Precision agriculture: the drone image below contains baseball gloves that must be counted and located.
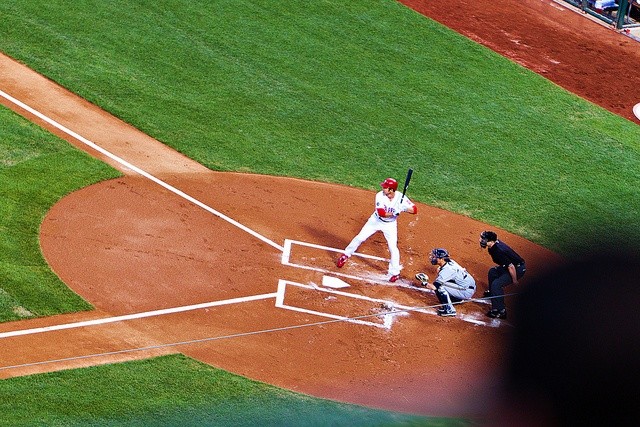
[415,272,430,286]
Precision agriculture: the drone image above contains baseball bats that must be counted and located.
[400,168,413,204]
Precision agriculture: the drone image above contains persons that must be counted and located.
[337,178,419,283]
[479,231,527,320]
[415,248,476,317]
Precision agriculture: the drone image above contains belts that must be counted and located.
[374,212,395,223]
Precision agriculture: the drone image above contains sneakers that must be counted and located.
[336,253,349,269]
[437,306,457,317]
[485,309,507,319]
[483,290,490,296]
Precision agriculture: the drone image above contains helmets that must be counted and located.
[379,177,399,191]
[478,230,498,249]
[429,248,452,266]
